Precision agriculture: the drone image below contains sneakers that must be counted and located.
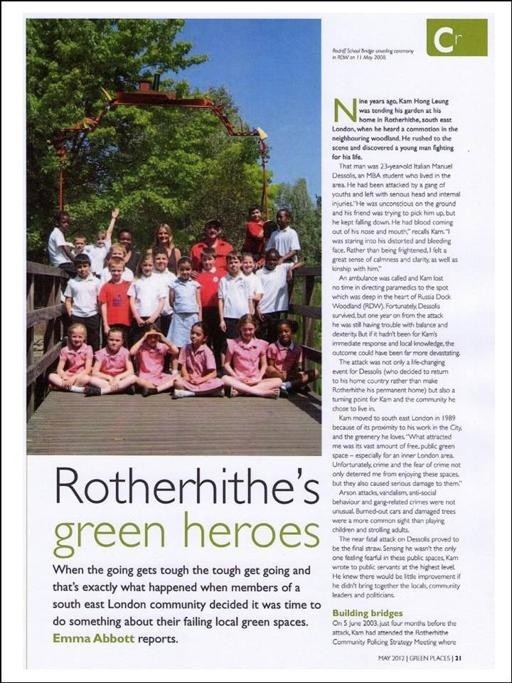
[281,384,289,397]
[172,389,186,399]
[84,387,101,396]
[126,385,136,395]
[229,388,239,397]
[215,389,225,396]
[143,387,158,397]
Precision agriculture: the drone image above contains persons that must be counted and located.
[173,321,225,399]
[258,222,277,252]
[153,247,177,319]
[100,244,133,284]
[153,224,181,264]
[130,324,179,397]
[219,251,255,337]
[65,254,102,346]
[258,248,305,321]
[84,328,137,397]
[49,212,76,277]
[191,219,233,276]
[50,323,92,395]
[99,257,131,334]
[165,257,202,347]
[127,254,166,335]
[267,320,319,397]
[241,254,261,312]
[222,314,282,399]
[82,208,119,274]
[118,230,140,271]
[264,210,300,265]
[73,234,87,255]
[241,205,267,252]
[191,248,226,322]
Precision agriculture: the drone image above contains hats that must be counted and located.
[204,218,221,226]
